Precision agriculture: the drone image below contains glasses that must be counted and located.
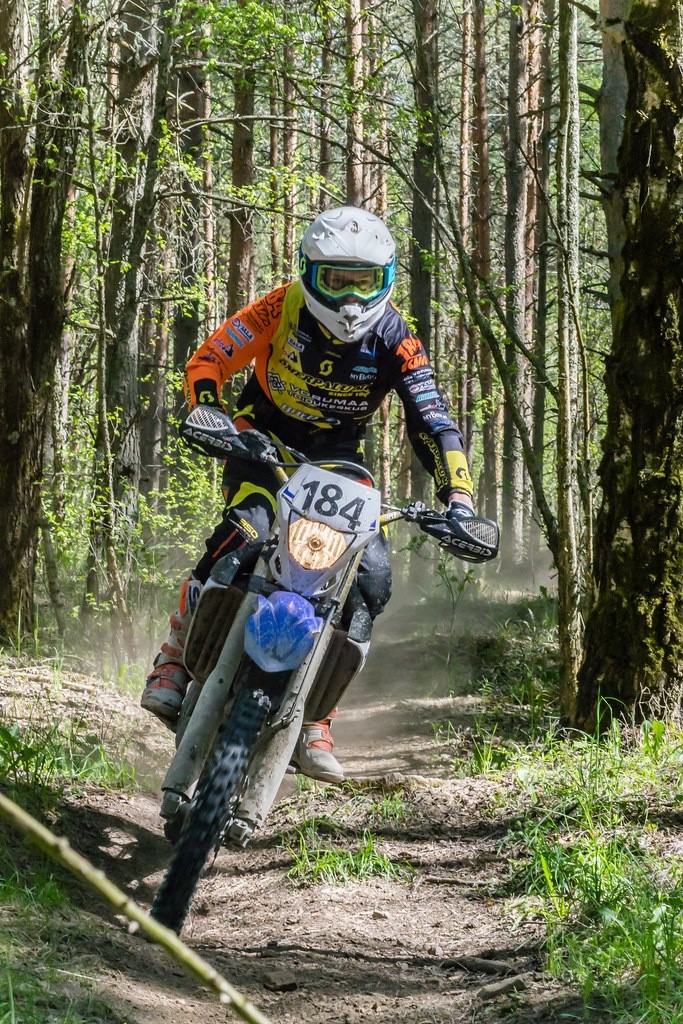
[298,247,396,303]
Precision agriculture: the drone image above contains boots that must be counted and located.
[291,640,371,784]
[140,573,207,719]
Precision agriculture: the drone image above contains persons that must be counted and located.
[141,206,476,784]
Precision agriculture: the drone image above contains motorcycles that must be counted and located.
[132,404,500,942]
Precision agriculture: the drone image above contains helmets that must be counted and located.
[298,206,397,342]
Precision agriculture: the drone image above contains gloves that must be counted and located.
[438,499,478,525]
